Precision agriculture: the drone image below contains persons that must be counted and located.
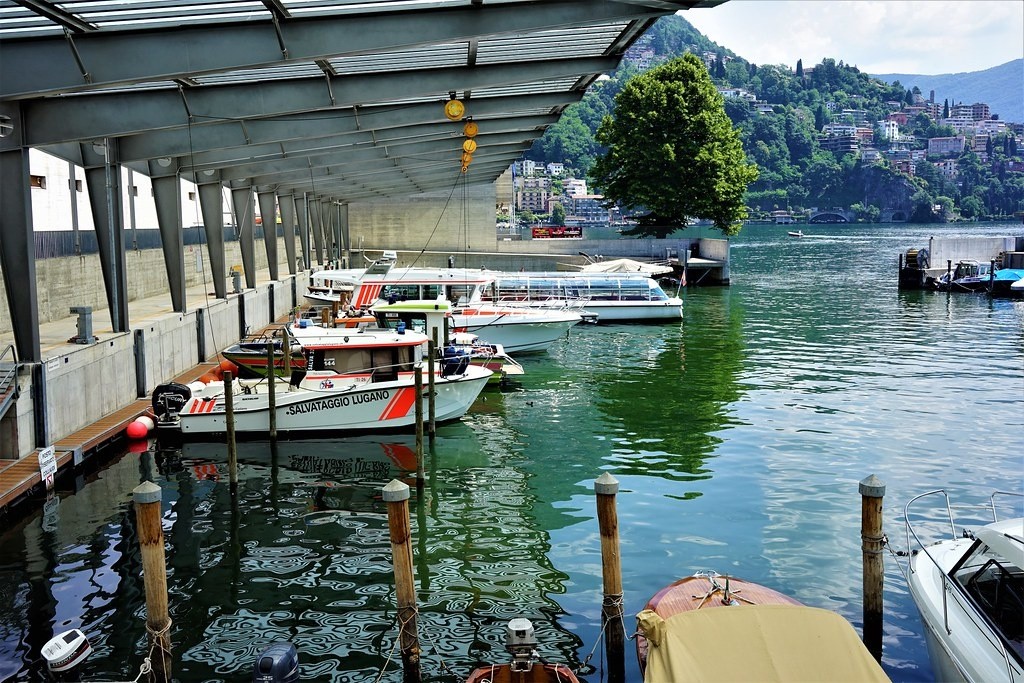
[966,266,970,275]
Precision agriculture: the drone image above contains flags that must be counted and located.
[682,270,686,286]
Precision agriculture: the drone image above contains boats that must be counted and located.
[634,570,894,683]
[152,304,495,440]
[463,617,583,683]
[218,252,688,387]
[532,226,583,239]
[787,230,805,237]
[895,486,1024,683]
